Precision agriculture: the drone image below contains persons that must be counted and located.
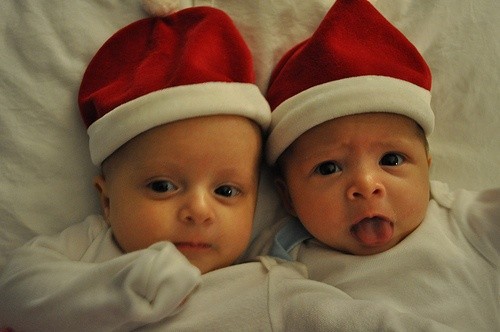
[234,0,499,332]
[2,0,456,332]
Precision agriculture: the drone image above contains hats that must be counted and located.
[78,0,273,167]
[265,0,436,166]
